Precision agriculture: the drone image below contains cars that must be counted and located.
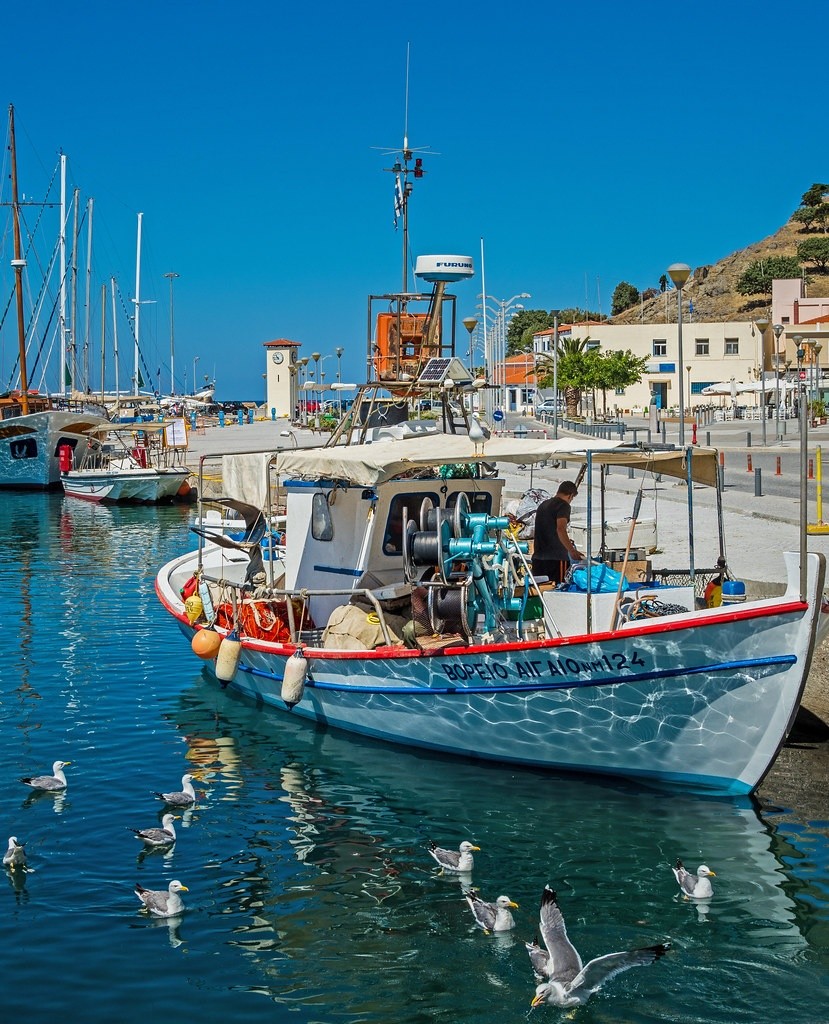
[206,405,222,414]
[302,401,319,413]
[319,400,346,410]
[415,400,431,410]
[536,401,566,414]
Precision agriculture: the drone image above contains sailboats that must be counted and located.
[7,145,109,417]
[0,102,55,419]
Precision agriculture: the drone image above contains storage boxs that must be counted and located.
[613,560,652,582]
[499,579,556,621]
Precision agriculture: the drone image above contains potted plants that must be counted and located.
[807,400,828,427]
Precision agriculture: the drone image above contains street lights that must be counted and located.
[792,336,823,433]
[686,366,692,412]
[473,292,531,438]
[666,263,693,446]
[462,317,478,372]
[771,325,784,437]
[288,347,345,420]
[755,319,771,446]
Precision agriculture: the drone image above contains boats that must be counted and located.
[60,422,192,504]
[153,429,826,798]
[0,398,109,483]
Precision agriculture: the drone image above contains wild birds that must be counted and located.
[671,856,717,900]
[18,761,71,791]
[126,813,182,846]
[2,834,27,868]
[469,410,492,457]
[426,839,480,871]
[464,890,520,936]
[132,880,189,916]
[150,773,197,807]
[526,884,672,1020]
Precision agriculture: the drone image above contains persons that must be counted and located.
[531,481,585,584]
[384,516,404,554]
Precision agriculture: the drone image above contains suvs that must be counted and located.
[223,401,248,415]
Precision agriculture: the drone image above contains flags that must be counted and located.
[393,160,404,230]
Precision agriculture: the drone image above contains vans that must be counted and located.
[431,400,463,417]
